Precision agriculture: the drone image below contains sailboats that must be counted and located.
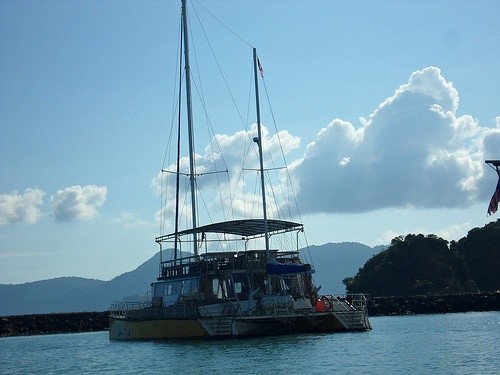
[108,1,374,342]
